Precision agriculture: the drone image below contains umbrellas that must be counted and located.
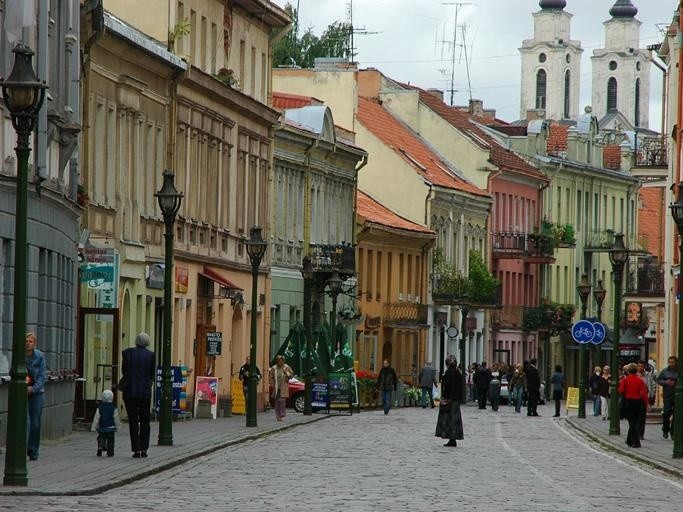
[275,318,353,375]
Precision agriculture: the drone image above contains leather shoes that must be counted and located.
[443,440,457,447]
[141,452,148,458]
[132,452,140,457]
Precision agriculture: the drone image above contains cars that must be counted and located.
[500,373,546,406]
[269,377,305,412]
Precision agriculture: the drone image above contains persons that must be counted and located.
[600,365,612,422]
[459,362,526,411]
[239,356,262,413]
[551,365,567,417]
[376,359,397,414]
[268,355,295,420]
[589,367,604,417]
[436,354,464,447]
[21,333,47,461]
[617,363,651,447]
[527,359,541,417]
[120,333,157,458]
[636,361,651,441]
[419,361,438,408]
[656,356,678,441]
[92,390,120,457]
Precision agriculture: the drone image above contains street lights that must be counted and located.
[577,271,587,418]
[593,279,607,415]
[241,223,268,428]
[325,266,341,377]
[667,181,682,463]
[575,272,591,419]
[670,180,683,460]
[593,279,605,411]
[325,268,343,415]
[245,225,268,428]
[608,232,629,437]
[607,229,626,438]
